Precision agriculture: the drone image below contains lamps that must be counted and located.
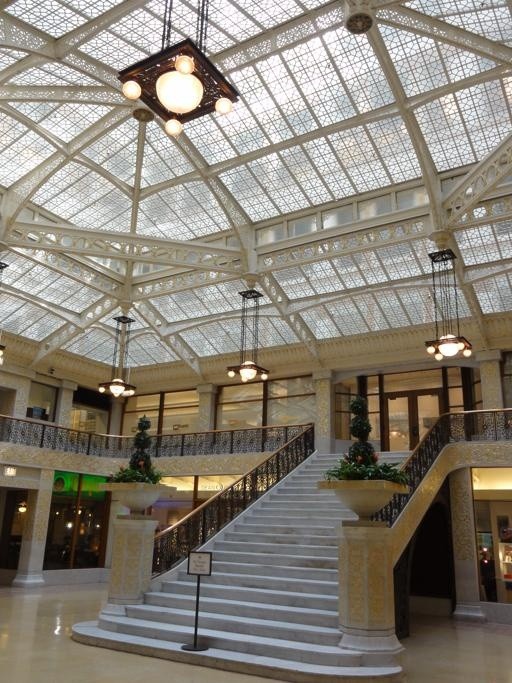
[117,0,240,138]
[18,501,30,514]
[425,248,473,362]
[98,315,137,399]
[226,289,270,383]
[0,261,9,366]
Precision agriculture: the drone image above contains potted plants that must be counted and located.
[317,394,411,527]
[97,413,165,521]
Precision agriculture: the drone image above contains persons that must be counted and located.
[480,547,494,602]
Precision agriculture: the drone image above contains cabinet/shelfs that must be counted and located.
[498,542,512,582]
